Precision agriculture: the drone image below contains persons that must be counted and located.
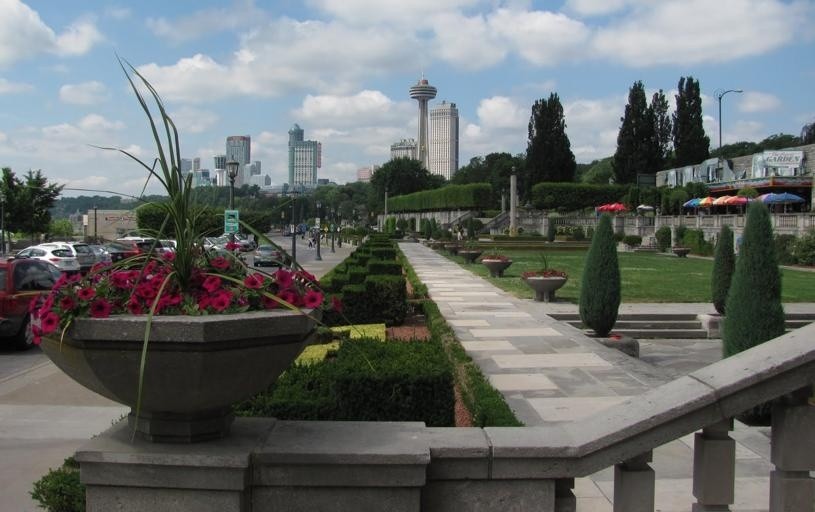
[41,231,48,243]
[448,224,464,241]
[302,233,323,248]
[278,257,291,271]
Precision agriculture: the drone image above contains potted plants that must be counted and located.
[563,212,641,357]
[697,221,733,339]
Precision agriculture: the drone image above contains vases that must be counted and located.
[428,240,566,304]
[672,247,691,257]
[23,305,335,444]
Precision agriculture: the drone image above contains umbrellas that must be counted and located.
[597,203,627,212]
[637,204,653,210]
[682,192,805,215]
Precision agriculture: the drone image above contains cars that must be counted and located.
[1,231,254,356]
[254,243,291,269]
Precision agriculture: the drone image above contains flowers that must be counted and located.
[3,241,348,345]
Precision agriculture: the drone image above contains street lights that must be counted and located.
[717,88,744,178]
[314,199,323,261]
[226,154,241,253]
[285,186,302,274]
